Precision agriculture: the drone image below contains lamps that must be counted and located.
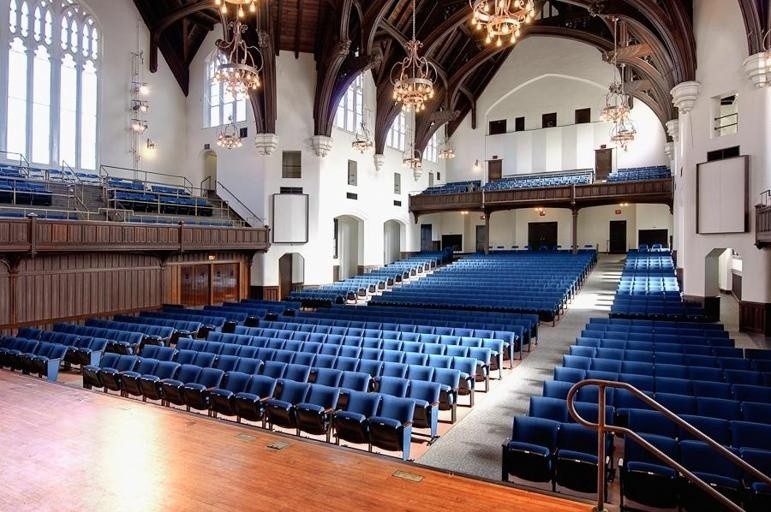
[611,63,637,151]
[469,0,535,48]
[214,0,264,99]
[217,116,242,150]
[389,1,438,114]
[402,109,422,168]
[438,122,455,159]
[130,81,155,160]
[352,88,373,153]
[600,18,630,123]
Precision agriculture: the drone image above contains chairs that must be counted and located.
[0,162,233,226]
[422,166,670,194]
